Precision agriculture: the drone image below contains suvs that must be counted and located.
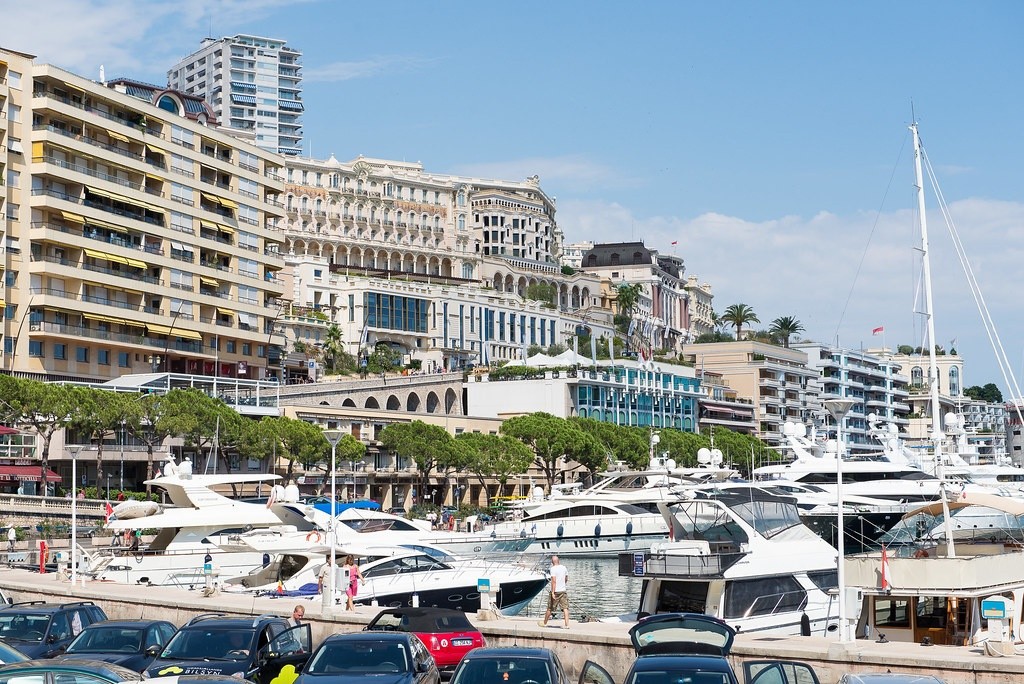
[140,611,316,682]
[0,599,112,658]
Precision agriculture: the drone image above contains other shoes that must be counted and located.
[562,625,570,629]
[538,621,547,627]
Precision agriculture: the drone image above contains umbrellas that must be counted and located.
[0,425,20,435]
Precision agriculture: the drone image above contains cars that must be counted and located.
[834,665,947,684]
[448,642,572,684]
[287,630,442,684]
[359,606,487,675]
[576,612,824,684]
[50,619,179,678]
[110,673,259,683]
[0,642,34,670]
[0,657,145,684]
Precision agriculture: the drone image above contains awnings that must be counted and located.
[0,466,62,481]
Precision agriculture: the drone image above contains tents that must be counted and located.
[312,499,381,516]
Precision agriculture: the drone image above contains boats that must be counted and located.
[7,94,1024,655]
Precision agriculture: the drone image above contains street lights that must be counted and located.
[61,444,89,584]
[319,428,346,609]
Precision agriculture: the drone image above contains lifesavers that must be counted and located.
[266,487,276,509]
[450,516,454,530]
[306,531,321,543]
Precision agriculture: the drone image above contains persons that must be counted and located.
[65,487,88,498]
[18,484,24,495]
[318,553,366,613]
[285,605,306,654]
[117,489,125,501]
[536,555,571,629]
[426,510,450,531]
[6,524,18,552]
[110,529,143,554]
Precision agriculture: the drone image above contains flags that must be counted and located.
[277,575,286,595]
[668,518,676,542]
[154,468,162,480]
[105,501,114,524]
[873,326,883,336]
[881,544,892,594]
[672,241,677,245]
